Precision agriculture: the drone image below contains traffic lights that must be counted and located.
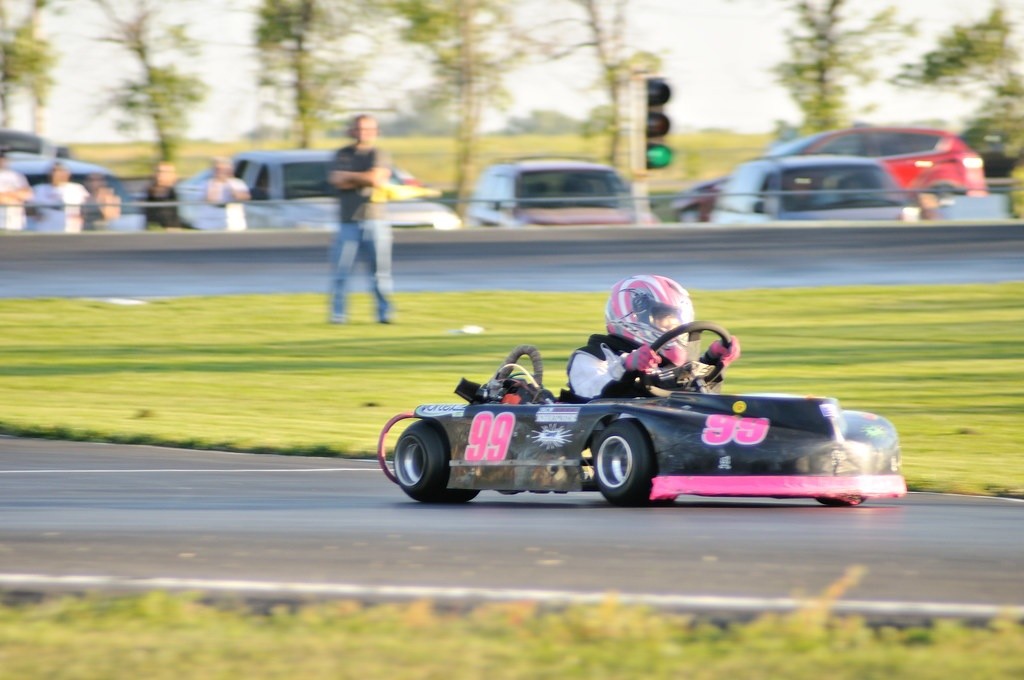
[643,75,675,171]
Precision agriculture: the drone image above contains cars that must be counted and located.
[171,150,464,233]
[466,157,662,227]
[0,128,146,234]
[709,153,934,222]
[665,125,988,233]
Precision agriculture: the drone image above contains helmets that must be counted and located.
[605,274,694,366]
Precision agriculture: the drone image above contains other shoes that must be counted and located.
[380,313,394,323]
[331,313,345,324]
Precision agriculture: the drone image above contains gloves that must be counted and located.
[625,344,662,373]
[706,335,740,367]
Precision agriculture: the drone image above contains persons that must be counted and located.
[567,274,741,404]
[145,163,197,230]
[327,115,400,325]
[80,174,121,230]
[835,174,882,209]
[24,163,90,233]
[0,153,34,230]
[204,158,251,207]
